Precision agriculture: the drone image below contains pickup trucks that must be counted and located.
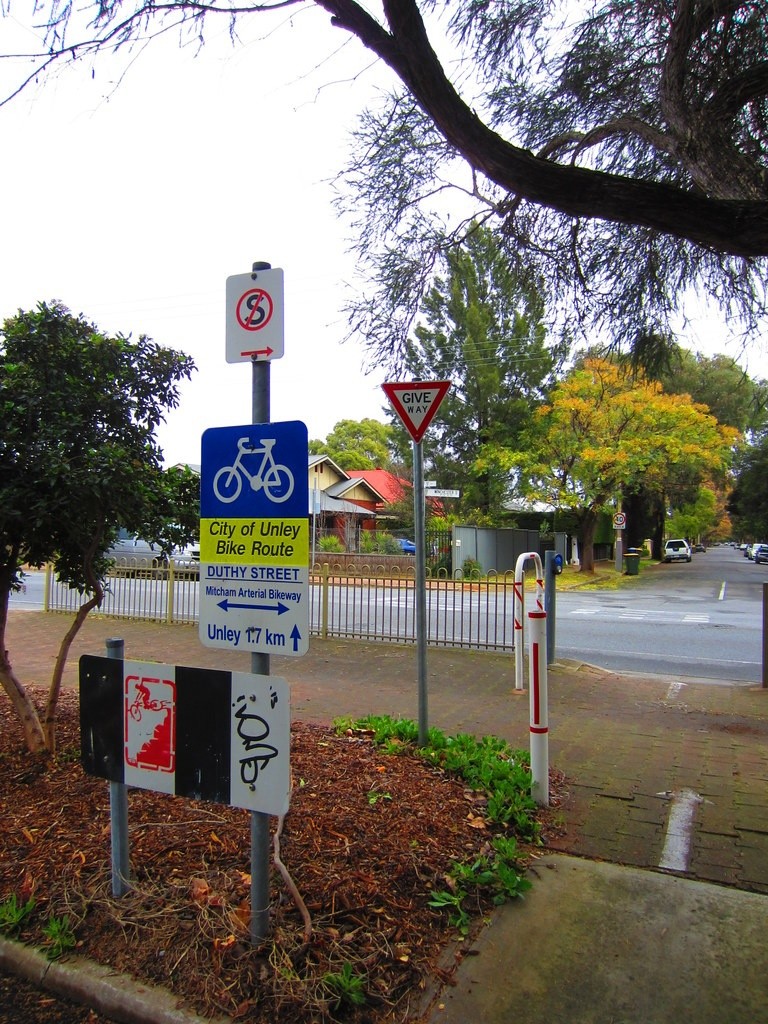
[663,540,691,562]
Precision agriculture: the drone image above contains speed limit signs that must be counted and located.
[612,512,626,530]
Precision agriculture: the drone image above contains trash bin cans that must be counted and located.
[623,553,640,576]
[628,548,643,558]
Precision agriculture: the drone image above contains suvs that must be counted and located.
[755,545,768,563]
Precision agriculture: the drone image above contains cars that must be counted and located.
[695,544,706,552]
[734,543,740,550]
[748,543,768,560]
[103,523,200,580]
[724,542,728,545]
[709,542,722,547]
[740,544,747,550]
[729,542,735,546]
[394,539,433,556]
[744,544,753,557]
